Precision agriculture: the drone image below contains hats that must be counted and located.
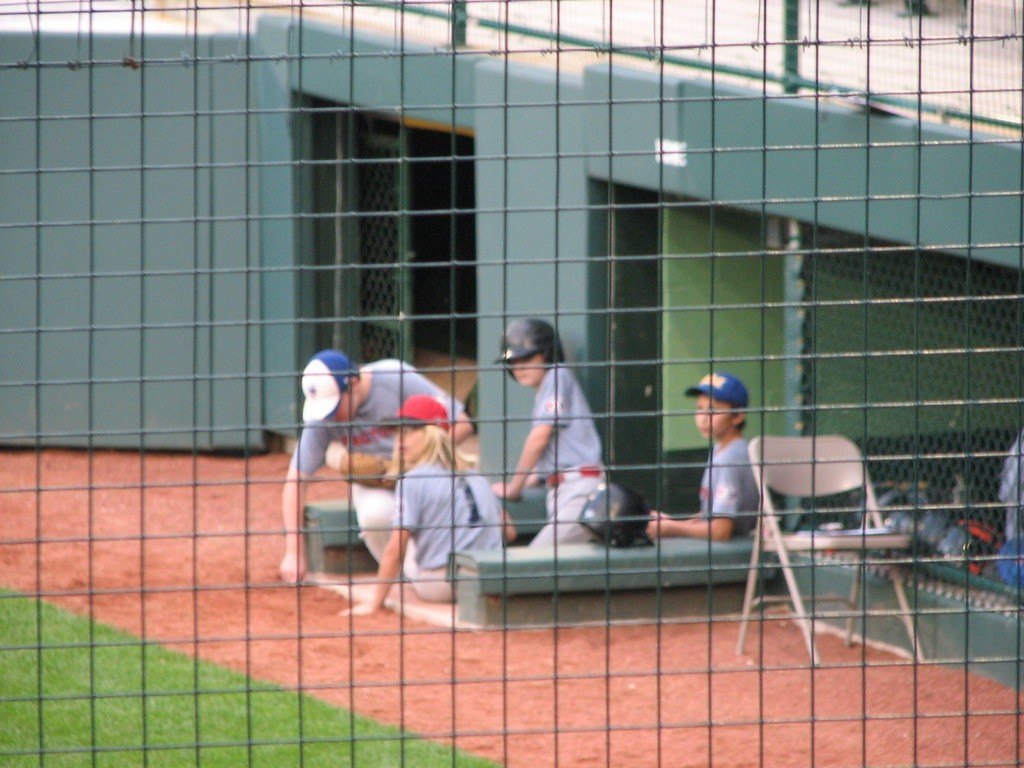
[385,395,450,433]
[301,349,355,423]
[684,371,748,408]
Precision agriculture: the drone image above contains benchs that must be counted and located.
[303,484,546,578]
[438,534,776,629]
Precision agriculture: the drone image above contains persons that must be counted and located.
[490,318,608,547]
[281,350,473,586]
[339,395,516,617]
[646,371,759,542]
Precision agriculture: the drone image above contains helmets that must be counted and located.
[492,317,564,379]
[578,483,654,548]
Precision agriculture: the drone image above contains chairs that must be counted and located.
[734,434,928,668]
